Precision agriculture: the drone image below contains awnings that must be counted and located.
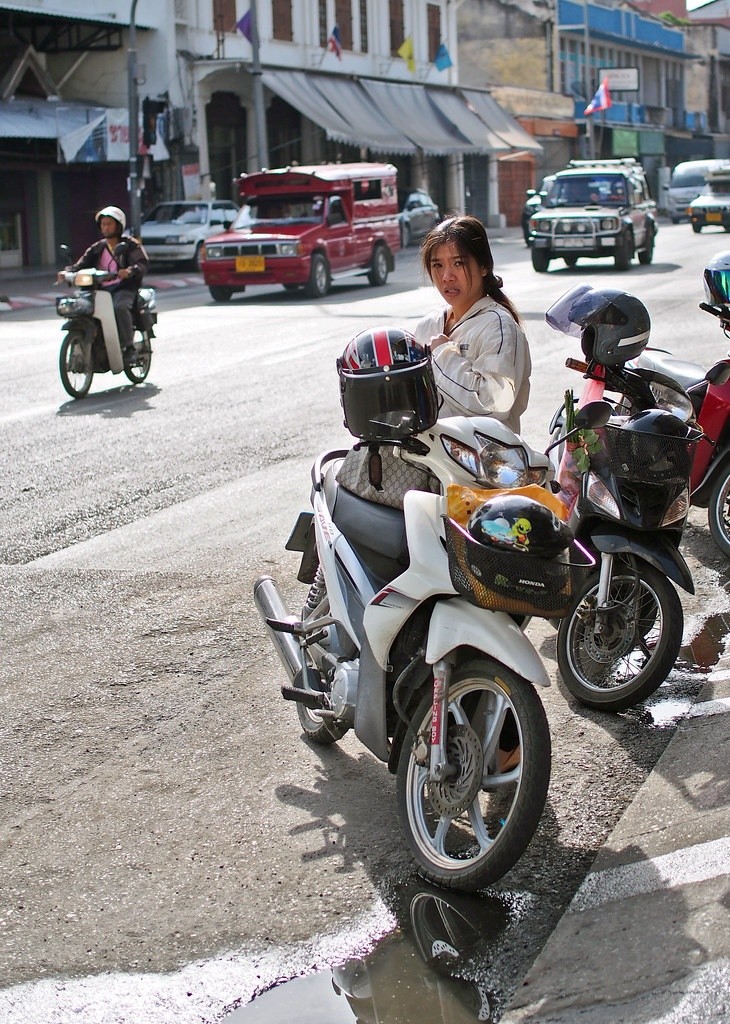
[246,67,544,157]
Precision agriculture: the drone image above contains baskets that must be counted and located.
[55,292,94,319]
[604,422,706,487]
[440,512,596,619]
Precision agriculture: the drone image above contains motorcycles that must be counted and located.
[253,399,615,893]
[53,240,159,400]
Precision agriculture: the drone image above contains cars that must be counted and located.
[395,186,440,250]
[129,199,241,274]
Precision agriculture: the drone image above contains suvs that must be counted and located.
[199,162,400,303]
[519,155,658,274]
[686,166,730,233]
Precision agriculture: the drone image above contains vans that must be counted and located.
[662,159,729,224]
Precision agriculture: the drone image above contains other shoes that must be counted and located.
[497,746,521,774]
[123,346,136,361]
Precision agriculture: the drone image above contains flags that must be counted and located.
[238,10,251,41]
[584,76,612,115]
[329,26,340,57]
[398,36,416,73]
[435,44,452,72]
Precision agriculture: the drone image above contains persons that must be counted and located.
[413,216,532,773]
[58,206,149,360]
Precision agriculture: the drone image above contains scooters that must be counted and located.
[612,302,730,559]
[546,359,719,715]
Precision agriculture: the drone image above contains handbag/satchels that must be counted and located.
[334,436,441,509]
[449,483,571,617]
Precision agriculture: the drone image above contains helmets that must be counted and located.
[568,289,650,364]
[336,326,439,441]
[465,495,574,600]
[95,206,126,232]
[702,251,730,307]
[614,410,705,483]
